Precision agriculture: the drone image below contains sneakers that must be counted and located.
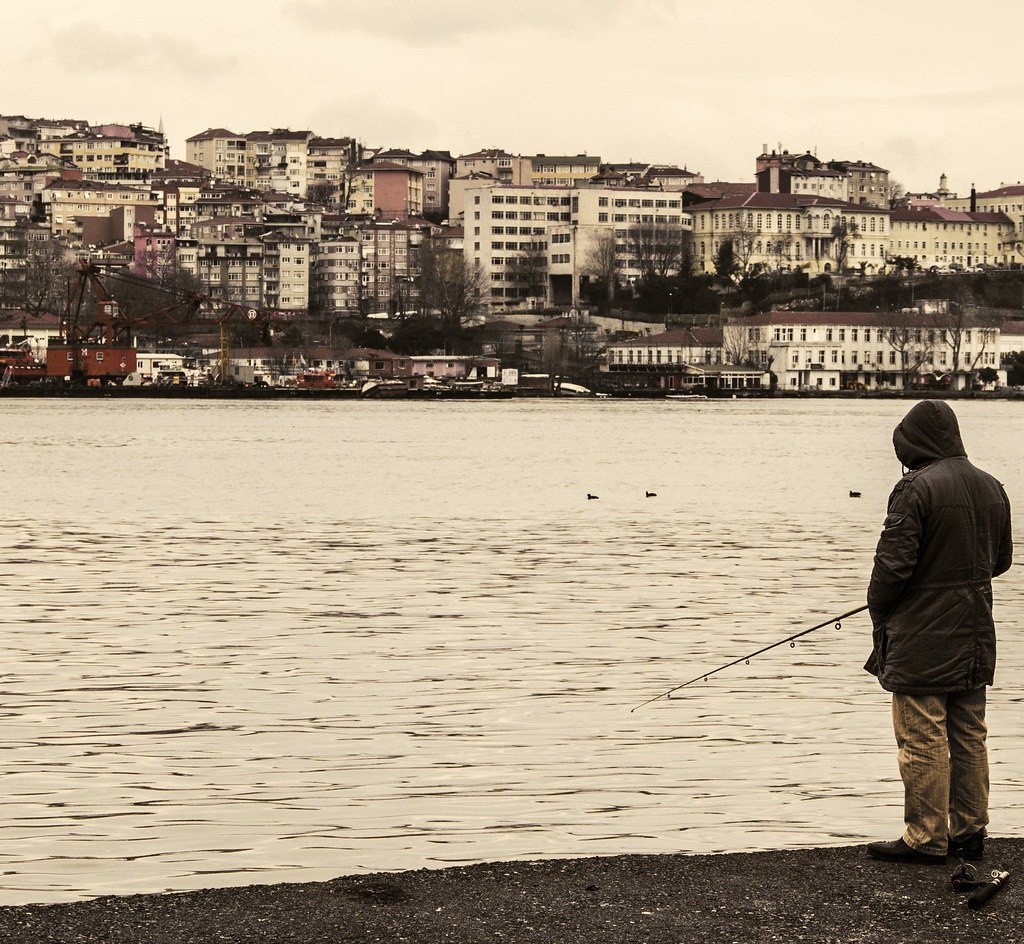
[866,836,948,864]
[947,832,984,860]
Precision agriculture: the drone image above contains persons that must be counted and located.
[864,400,1013,863]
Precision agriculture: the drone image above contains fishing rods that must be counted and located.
[630,604,868,714]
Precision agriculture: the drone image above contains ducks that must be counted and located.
[587,490,861,500]
